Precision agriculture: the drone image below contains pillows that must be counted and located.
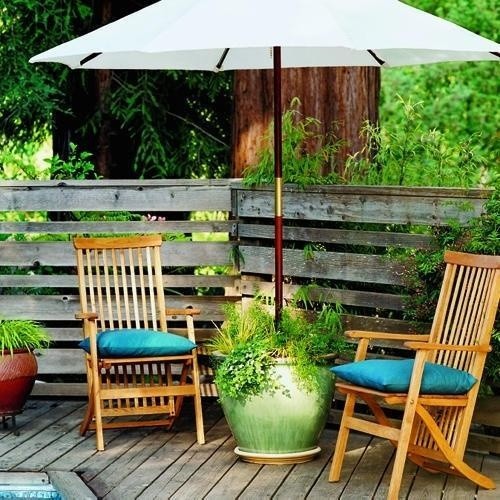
[78,330,198,357]
[331,358,478,396]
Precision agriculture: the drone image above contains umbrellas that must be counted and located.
[28,0,500,335]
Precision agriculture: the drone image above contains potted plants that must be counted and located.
[204,285,342,464]
[0,320,49,436]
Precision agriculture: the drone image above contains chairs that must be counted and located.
[72,236,207,452]
[329,251,500,496]
